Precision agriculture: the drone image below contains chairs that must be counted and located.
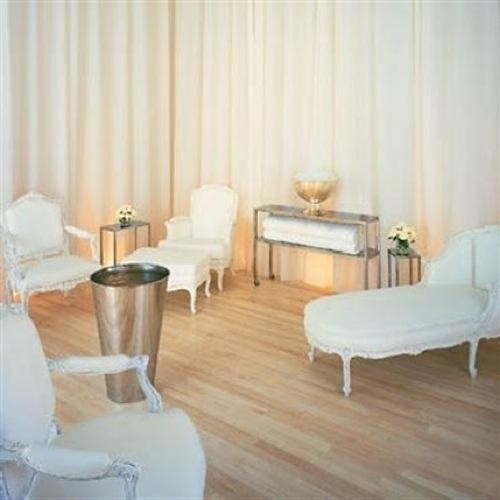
[302,224,500,398]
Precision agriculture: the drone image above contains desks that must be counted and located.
[387,248,422,288]
[252,203,381,290]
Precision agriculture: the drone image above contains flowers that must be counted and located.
[388,222,417,248]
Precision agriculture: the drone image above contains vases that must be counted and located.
[395,239,410,255]
